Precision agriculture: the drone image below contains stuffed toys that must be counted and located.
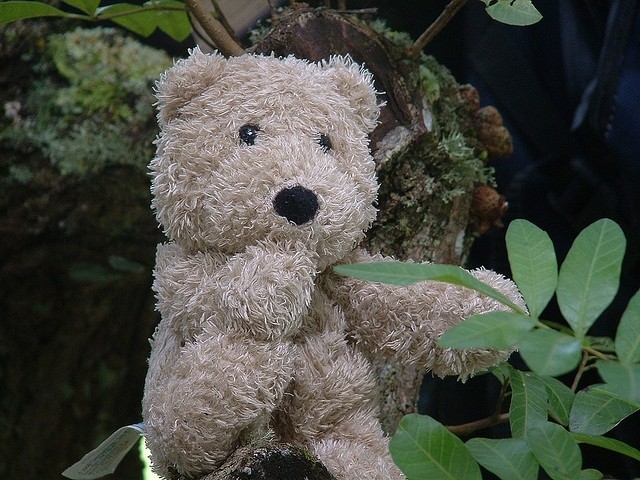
[142,44,528,480]
